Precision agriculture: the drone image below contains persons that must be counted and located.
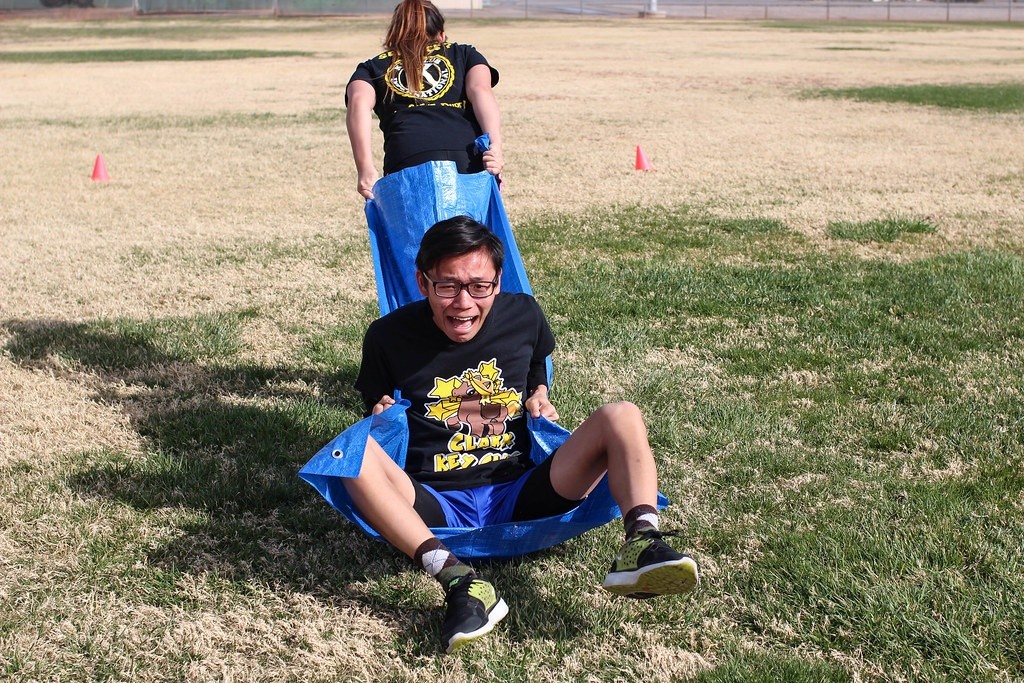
[345,0,504,200]
[341,215,699,655]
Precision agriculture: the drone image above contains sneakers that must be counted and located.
[602,528,699,600]
[440,572,509,653]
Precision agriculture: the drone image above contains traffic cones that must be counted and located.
[635,146,649,170]
[92,152,110,179]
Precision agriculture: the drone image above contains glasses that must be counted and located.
[422,272,498,299]
